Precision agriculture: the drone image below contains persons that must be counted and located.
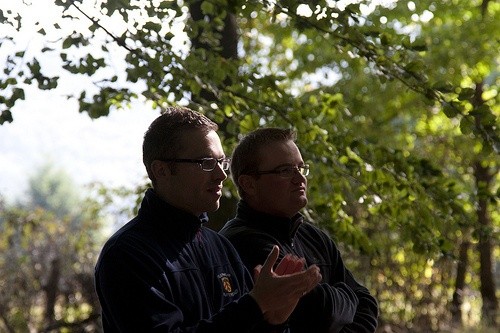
[94,107,323,333]
[216,128,379,333]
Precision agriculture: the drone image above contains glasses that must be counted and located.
[161,157,231,172]
[239,164,310,177]
